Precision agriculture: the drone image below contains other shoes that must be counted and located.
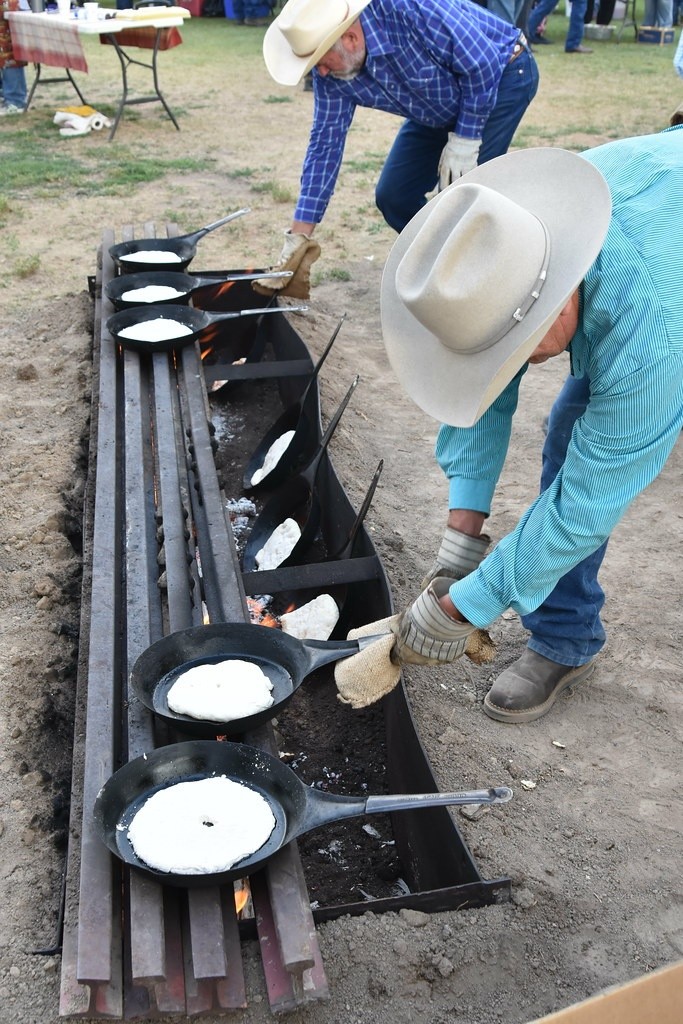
[565,44,594,53]
[531,36,553,44]
[244,16,276,25]
[232,18,243,26]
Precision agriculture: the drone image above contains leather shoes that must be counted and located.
[482,644,595,724]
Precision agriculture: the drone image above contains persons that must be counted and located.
[249,0,540,300]
[473,0,683,127]
[0,0,30,117]
[43,0,277,25]
[380,122,683,723]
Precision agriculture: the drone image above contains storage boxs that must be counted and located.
[585,25,615,39]
[639,25,675,43]
[178,0,270,18]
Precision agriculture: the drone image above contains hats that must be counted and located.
[263,0,374,86]
[380,146,612,430]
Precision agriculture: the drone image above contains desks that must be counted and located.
[3,7,190,142]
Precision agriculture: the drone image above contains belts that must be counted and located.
[507,32,528,60]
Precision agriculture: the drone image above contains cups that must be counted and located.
[58,0,70,15]
[83,3,98,21]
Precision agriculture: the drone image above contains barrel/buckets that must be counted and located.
[29,0,44,13]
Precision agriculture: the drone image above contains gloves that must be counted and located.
[250,229,321,301]
[389,576,478,669]
[421,526,493,594]
[437,131,482,192]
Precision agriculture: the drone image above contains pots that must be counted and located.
[105,270,294,311]
[242,313,344,491]
[130,622,392,739]
[108,207,252,272]
[293,458,384,614]
[241,375,358,574]
[106,304,311,353]
[91,739,514,890]
